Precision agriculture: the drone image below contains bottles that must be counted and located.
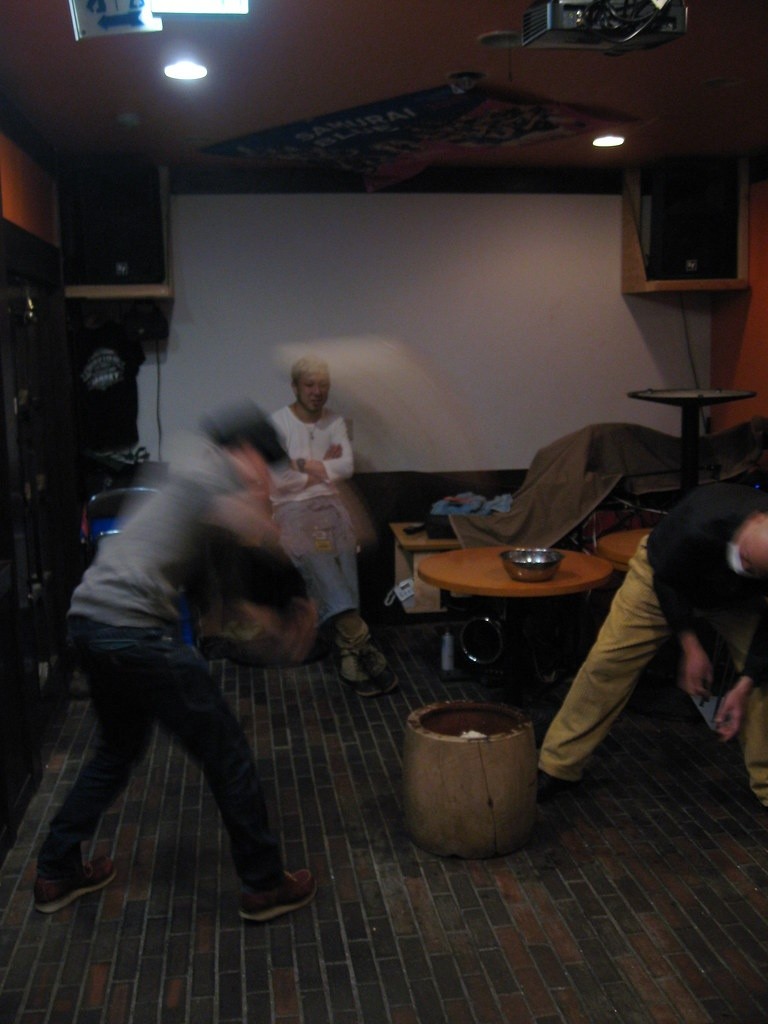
[439,627,456,670]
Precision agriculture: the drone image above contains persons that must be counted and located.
[264,351,400,696]
[534,477,768,814]
[36,392,316,921]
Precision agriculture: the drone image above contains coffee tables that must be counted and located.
[390,521,476,615]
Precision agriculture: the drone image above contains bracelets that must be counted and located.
[296,458,305,472]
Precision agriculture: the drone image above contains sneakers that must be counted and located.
[33,856,116,914]
[236,870,315,922]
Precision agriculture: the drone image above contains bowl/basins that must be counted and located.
[498,547,566,582]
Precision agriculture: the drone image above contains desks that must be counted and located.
[418,528,674,705]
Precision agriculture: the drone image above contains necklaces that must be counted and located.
[294,402,319,440]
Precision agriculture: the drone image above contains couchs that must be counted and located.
[216,468,535,624]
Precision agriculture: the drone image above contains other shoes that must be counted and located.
[339,673,380,697]
[536,769,574,803]
[374,663,400,694]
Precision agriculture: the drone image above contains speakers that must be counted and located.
[635,154,738,280]
[55,154,167,288]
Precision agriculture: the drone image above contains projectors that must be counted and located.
[521,0,685,55]
